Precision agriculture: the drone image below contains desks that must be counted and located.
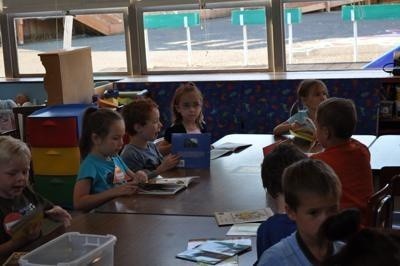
[0,132,400,266]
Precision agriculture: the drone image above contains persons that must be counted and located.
[256,158,347,266]
[73,106,149,211]
[0,135,73,255]
[307,96,374,227]
[272,79,330,137]
[256,143,311,259]
[120,98,180,179]
[164,82,208,144]
[316,208,400,266]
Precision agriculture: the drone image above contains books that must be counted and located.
[131,174,201,195]
[210,141,252,161]
[171,131,211,169]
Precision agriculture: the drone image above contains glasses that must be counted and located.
[174,101,203,111]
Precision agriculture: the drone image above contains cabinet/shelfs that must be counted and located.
[23,103,99,211]
